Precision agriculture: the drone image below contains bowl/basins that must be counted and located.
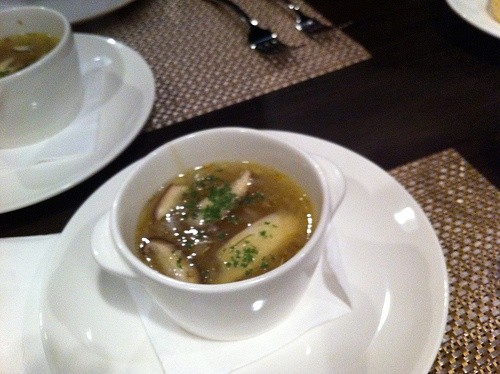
[91,125,347,341]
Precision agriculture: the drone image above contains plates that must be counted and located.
[0,32,155,212]
[0,1,137,24]
[38,130,448,374]
[445,0,500,38]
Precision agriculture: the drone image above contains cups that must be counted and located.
[0,7,85,150]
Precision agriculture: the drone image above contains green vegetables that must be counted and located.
[171,166,279,277]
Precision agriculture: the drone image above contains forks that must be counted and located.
[275,0,346,35]
[220,0,306,56]
